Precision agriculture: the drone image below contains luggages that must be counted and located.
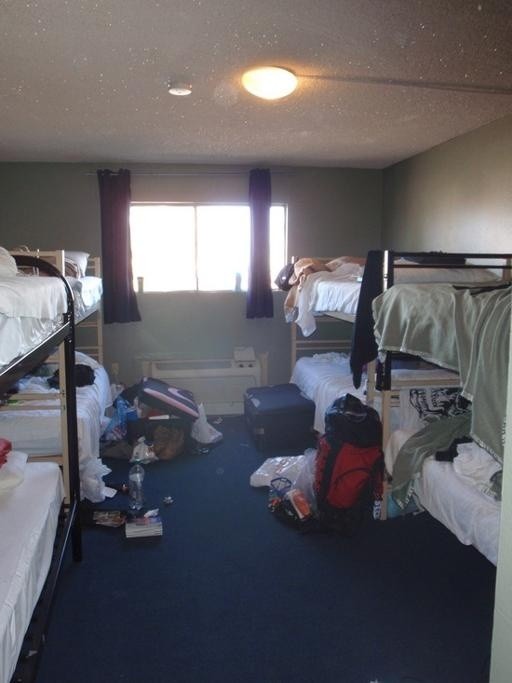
[243,382,315,455]
[124,377,200,450]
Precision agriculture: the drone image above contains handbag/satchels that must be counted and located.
[324,395,380,449]
[312,434,385,510]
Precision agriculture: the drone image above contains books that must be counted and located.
[92,503,163,539]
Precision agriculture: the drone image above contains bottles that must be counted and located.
[128,458,146,510]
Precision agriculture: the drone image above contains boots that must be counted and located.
[151,425,188,462]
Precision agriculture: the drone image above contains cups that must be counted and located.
[122,407,138,422]
